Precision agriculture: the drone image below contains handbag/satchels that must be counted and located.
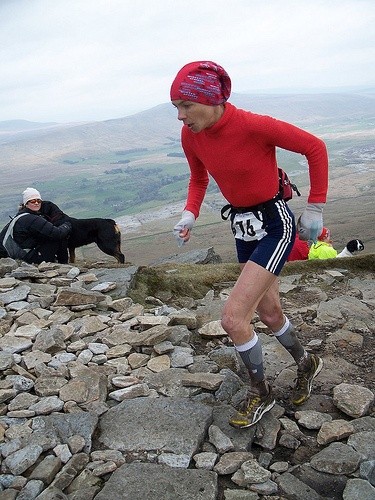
[221,167,301,221]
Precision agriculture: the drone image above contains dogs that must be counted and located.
[336,239,364,257]
[38,201,125,264]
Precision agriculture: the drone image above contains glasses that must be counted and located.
[28,199,42,204]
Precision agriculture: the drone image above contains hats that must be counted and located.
[22,187,42,206]
[347,239,364,253]
[170,60,232,106]
[317,226,331,242]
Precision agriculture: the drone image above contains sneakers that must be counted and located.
[228,383,277,429]
[292,352,325,406]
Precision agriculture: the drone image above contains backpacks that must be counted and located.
[0,212,41,263]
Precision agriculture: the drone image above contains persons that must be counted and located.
[170,61,328,428]
[285,227,338,261]
[9,187,72,264]
